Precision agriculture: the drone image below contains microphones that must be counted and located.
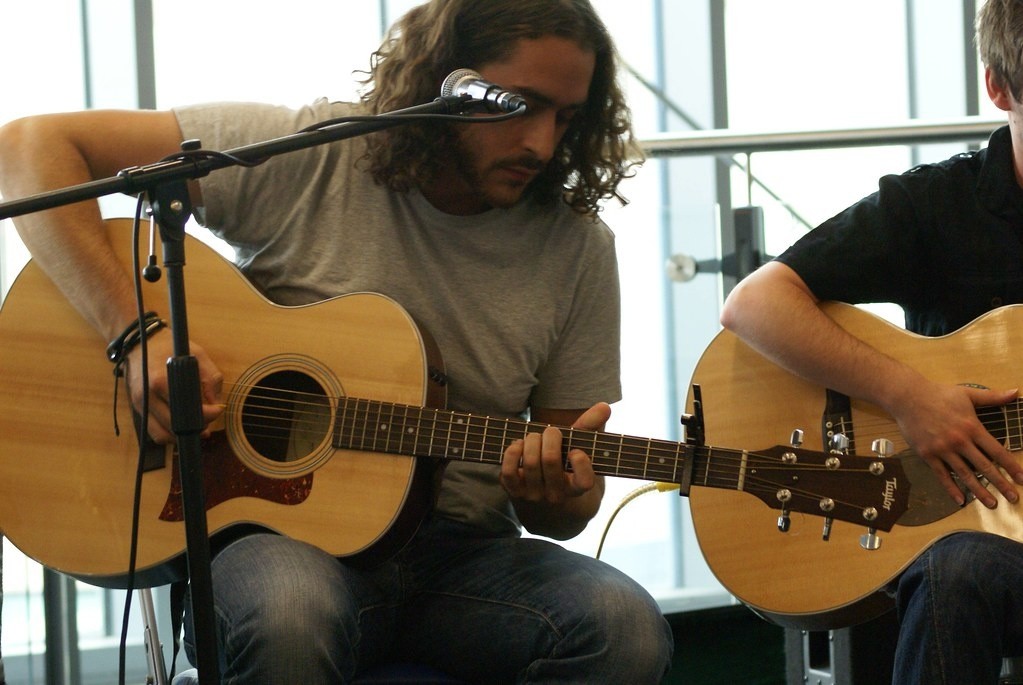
[436,67,528,114]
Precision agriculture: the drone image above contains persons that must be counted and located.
[721,0,1023,685]
[0,0,672,685]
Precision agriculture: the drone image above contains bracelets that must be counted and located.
[106,311,166,438]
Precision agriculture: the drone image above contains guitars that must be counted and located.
[0,217,912,590]
[681,300,1023,633]
